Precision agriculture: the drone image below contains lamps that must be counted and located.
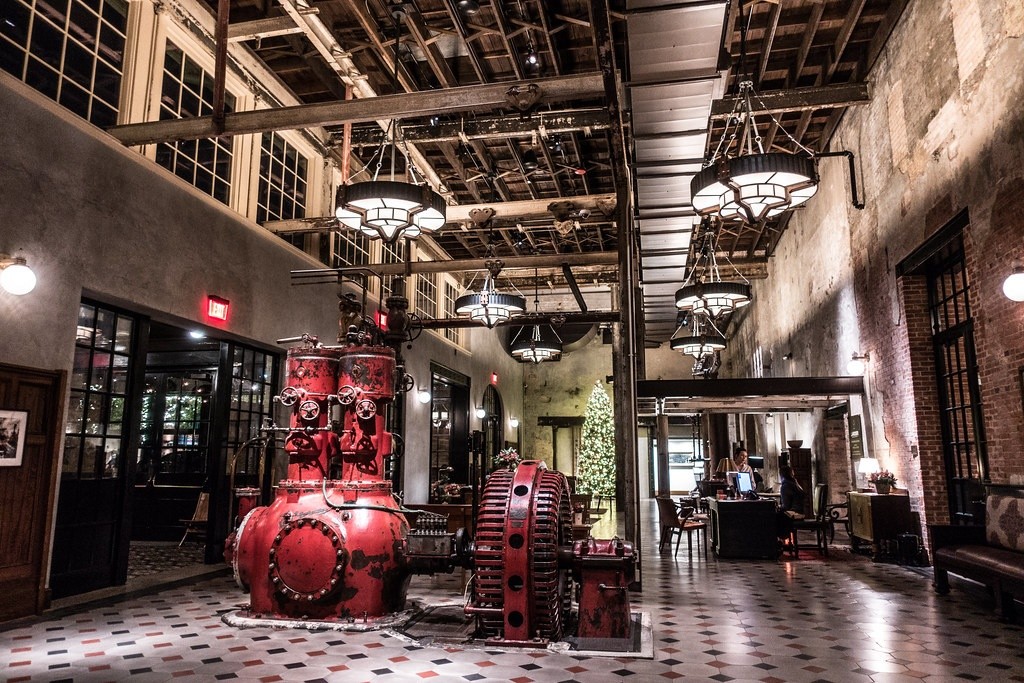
[1002,259,1024,303]
[334,6,447,246]
[675,218,753,320]
[508,267,563,366]
[417,386,431,404]
[453,158,527,330]
[847,351,870,376]
[0,247,37,296]
[510,416,518,427]
[476,405,485,418]
[669,310,727,361]
[689,0,820,227]
[857,456,881,491]
[766,414,774,424]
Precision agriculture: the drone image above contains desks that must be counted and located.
[847,489,910,562]
[706,495,779,561]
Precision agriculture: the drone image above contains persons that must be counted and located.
[108,453,118,467]
[717,448,757,491]
[774,465,808,551]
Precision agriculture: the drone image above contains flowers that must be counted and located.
[444,483,462,496]
[493,447,522,470]
[867,469,898,486]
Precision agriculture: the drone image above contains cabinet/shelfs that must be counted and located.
[781,447,815,518]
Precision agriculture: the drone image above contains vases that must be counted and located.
[875,482,891,495]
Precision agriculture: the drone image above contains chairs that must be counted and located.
[656,497,696,551]
[680,497,707,546]
[177,492,210,548]
[824,489,855,546]
[657,497,708,559]
[787,483,829,558]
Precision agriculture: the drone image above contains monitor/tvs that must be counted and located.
[736,472,753,495]
